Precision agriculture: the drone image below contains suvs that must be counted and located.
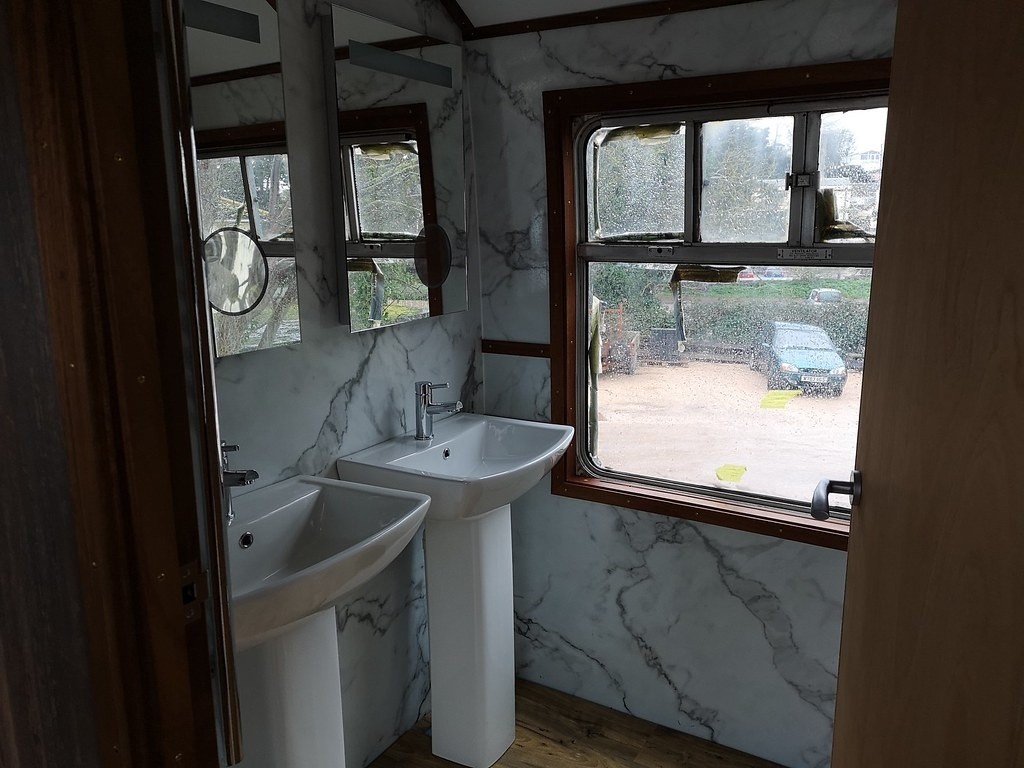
[764,266,785,277]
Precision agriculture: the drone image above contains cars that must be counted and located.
[749,321,847,397]
[809,288,845,308]
[739,267,754,279]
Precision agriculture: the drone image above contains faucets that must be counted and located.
[414,381,463,441]
[219,440,259,526]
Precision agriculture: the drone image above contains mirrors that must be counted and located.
[179,0,302,360]
[330,2,469,334]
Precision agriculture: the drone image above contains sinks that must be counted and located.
[221,476,433,636]
[336,412,574,521]
